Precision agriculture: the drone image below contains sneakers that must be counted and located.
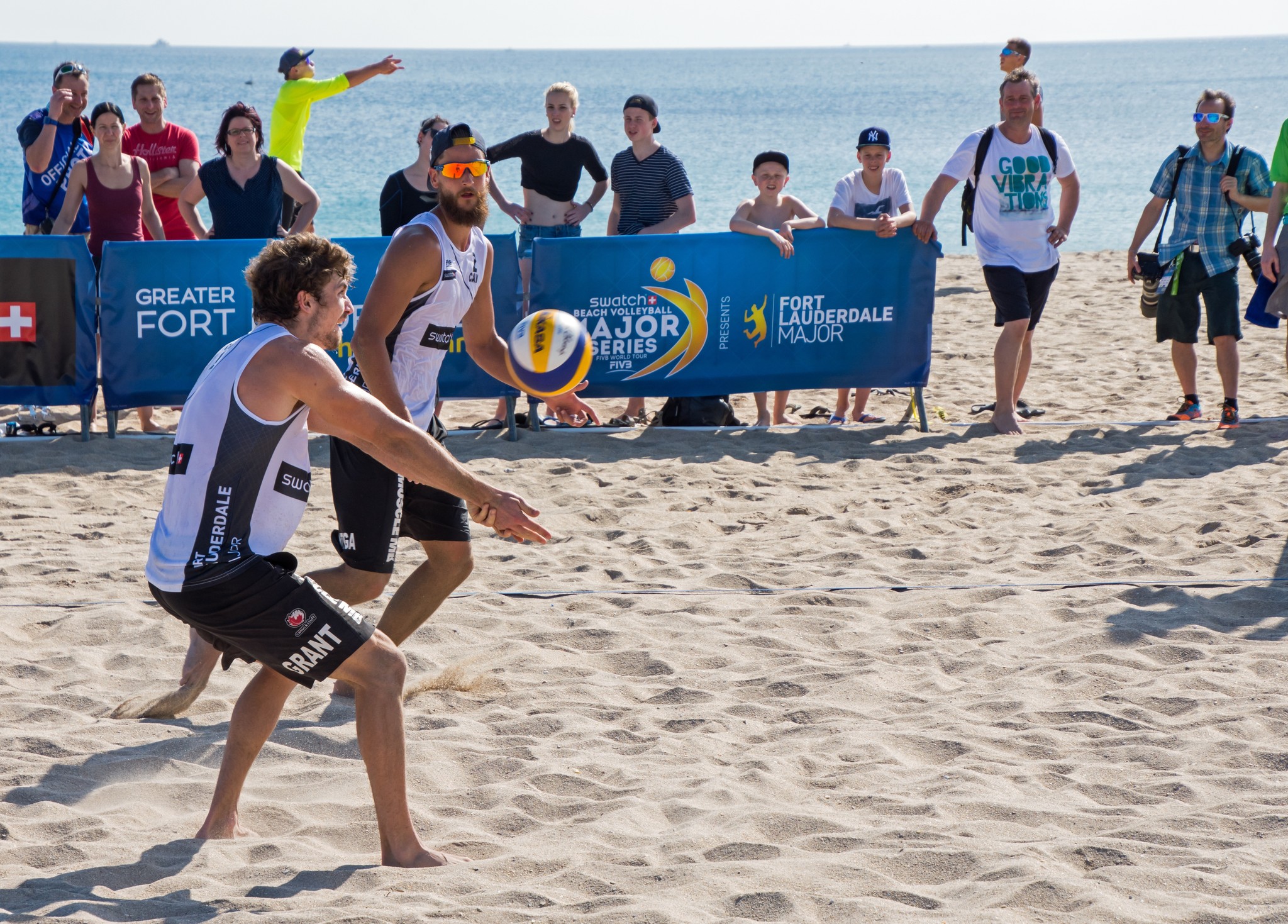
[1217,403,1240,429]
[1166,396,1202,420]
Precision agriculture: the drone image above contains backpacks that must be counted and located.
[961,123,1058,233]
[651,394,749,427]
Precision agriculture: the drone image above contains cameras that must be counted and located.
[1132,254,1168,319]
[1227,231,1262,285]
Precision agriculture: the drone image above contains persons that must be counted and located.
[50,102,169,433]
[179,102,320,239]
[16,60,94,244]
[998,38,1044,128]
[604,94,696,426]
[485,81,609,428]
[912,65,1081,435]
[729,150,826,427]
[380,117,451,418]
[179,123,600,698]
[1261,119,1288,366]
[826,125,917,425]
[1127,90,1274,430]
[267,46,406,233]
[121,72,200,411]
[145,231,552,868]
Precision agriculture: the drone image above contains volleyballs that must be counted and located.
[504,309,593,397]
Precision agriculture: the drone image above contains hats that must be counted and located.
[427,122,487,192]
[279,47,314,75]
[753,149,789,174]
[856,126,891,150]
[623,93,661,133]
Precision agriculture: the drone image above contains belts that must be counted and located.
[1186,243,1200,253]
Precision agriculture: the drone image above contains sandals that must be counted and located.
[603,407,647,427]
[615,410,663,427]
[18,404,36,421]
[36,405,56,421]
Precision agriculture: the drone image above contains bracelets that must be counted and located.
[584,201,593,212]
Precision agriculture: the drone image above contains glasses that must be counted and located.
[1002,48,1026,58]
[424,128,439,138]
[226,127,256,135]
[297,56,311,65]
[434,159,490,178]
[55,64,86,83]
[1192,112,1229,123]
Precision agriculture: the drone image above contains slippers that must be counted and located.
[457,419,503,430]
[785,404,802,414]
[799,405,832,417]
[971,402,997,412]
[859,414,886,423]
[1016,400,1045,418]
[514,411,545,425]
[14,423,38,437]
[38,423,65,436]
[828,411,847,424]
[538,415,591,426]
